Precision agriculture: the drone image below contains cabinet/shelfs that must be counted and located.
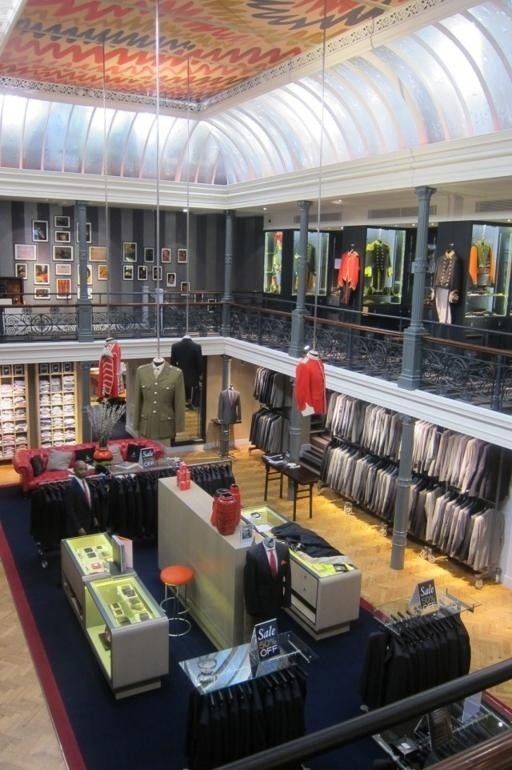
[238,504,361,642]
[35,362,78,448]
[0,364,31,461]
[82,569,170,700]
[59,531,136,632]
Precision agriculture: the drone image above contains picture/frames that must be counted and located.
[15,263,28,280]
[122,265,134,281]
[77,286,93,300]
[52,245,74,262]
[144,248,154,263]
[52,363,60,372]
[55,263,72,276]
[77,222,92,244]
[166,273,177,288]
[63,362,72,372]
[2,366,10,375]
[39,363,49,373]
[177,249,188,264]
[33,264,50,285]
[161,248,171,264]
[137,265,148,281]
[55,231,70,243]
[123,241,137,263]
[181,281,191,297]
[151,265,163,280]
[13,242,38,261]
[33,287,51,300]
[88,246,109,262]
[32,219,49,242]
[56,279,72,299]
[76,263,93,285]
[98,265,109,281]
[54,215,71,228]
[14,364,24,375]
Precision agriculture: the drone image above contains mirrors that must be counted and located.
[170,356,208,448]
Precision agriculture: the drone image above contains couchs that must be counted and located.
[11,437,165,498]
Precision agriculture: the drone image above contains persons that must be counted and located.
[65,460,100,537]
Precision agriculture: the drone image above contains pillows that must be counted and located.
[30,454,46,477]
[46,448,74,471]
[107,443,123,465]
[76,448,94,463]
[125,443,146,462]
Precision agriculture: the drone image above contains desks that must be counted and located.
[260,452,319,522]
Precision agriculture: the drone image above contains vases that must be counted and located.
[92,446,113,461]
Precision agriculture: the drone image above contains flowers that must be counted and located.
[82,401,126,445]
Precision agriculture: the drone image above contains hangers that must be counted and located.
[374,599,463,648]
[37,473,105,487]
[198,651,298,710]
[122,459,229,479]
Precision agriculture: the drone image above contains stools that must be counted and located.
[159,564,194,637]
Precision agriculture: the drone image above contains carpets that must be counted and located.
[0,480,512,770]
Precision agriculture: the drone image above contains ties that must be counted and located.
[268,551,278,578]
[82,479,91,507]
[229,391,233,408]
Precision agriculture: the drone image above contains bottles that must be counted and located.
[213,484,241,535]
